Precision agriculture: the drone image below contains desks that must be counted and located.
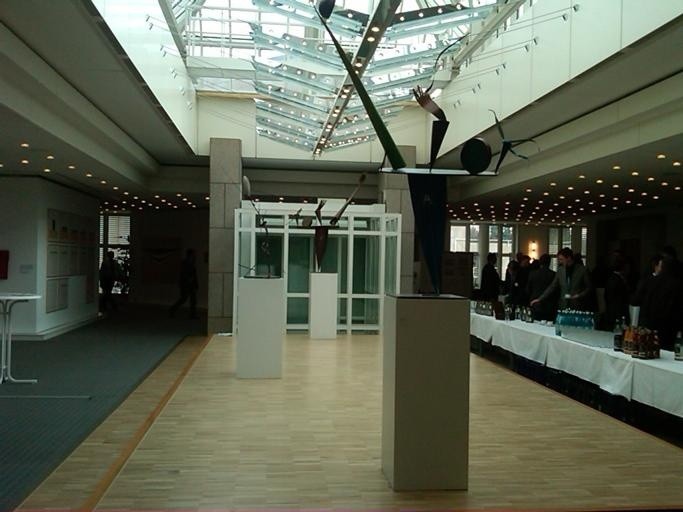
[470,301,683,448]
[0,293,41,385]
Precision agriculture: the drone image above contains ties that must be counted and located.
[566,268,571,290]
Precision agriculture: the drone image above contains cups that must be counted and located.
[474,300,534,323]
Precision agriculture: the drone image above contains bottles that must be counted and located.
[555,309,594,336]
[673,331,683,361]
[612,316,660,360]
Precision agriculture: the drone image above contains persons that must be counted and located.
[165,247,201,320]
[477,236,682,351]
[99,249,127,312]
